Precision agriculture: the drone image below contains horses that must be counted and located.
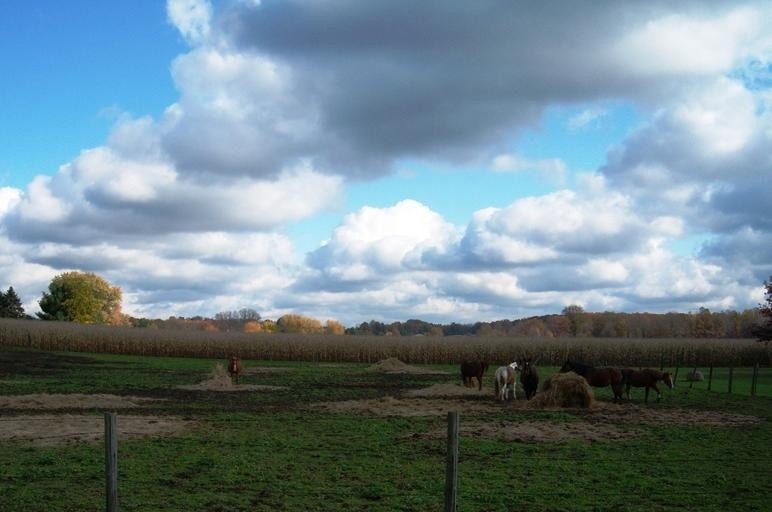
[519,359,539,400]
[558,359,675,404]
[494,360,519,402]
[226,352,243,385]
[460,357,487,391]
